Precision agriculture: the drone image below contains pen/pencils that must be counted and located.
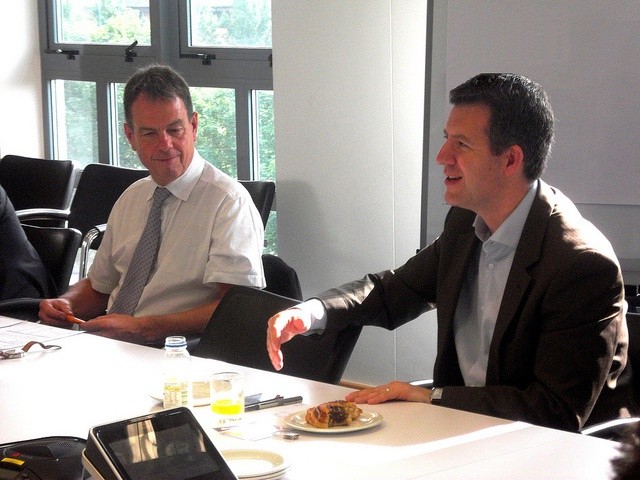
[244,395,302,411]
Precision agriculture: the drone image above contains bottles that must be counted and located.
[163,335,192,412]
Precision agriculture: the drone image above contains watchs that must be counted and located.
[0,341,62,359]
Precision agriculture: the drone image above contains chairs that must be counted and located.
[403,285,640,435]
[1,154,73,229]
[192,283,365,385]
[10,164,154,282]
[261,253,301,300]
[0,181,276,350]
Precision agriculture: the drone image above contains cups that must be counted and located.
[211,371,245,430]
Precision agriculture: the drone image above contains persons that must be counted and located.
[266,74,632,441]
[0,183,51,323]
[38,64,267,346]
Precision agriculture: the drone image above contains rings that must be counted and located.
[385,386,390,395]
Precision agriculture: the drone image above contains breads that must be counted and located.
[304,399,363,430]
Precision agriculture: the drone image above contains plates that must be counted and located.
[148,377,234,407]
[220,448,293,480]
[285,407,384,433]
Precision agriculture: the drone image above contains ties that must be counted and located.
[107,188,172,317]
